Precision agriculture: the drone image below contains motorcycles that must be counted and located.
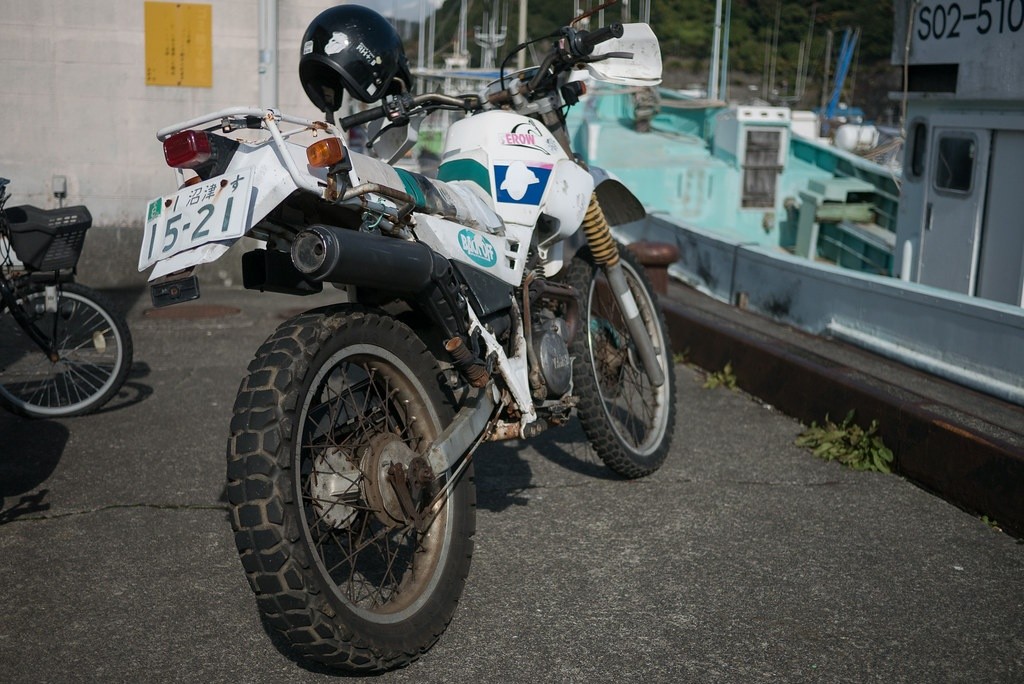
[135,0,678,678]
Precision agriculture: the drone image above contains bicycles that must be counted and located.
[1,175,134,419]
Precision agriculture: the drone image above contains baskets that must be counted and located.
[3,204,92,271]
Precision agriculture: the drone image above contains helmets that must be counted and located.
[299,4,413,112]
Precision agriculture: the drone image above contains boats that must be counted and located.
[416,0,1024,472]
